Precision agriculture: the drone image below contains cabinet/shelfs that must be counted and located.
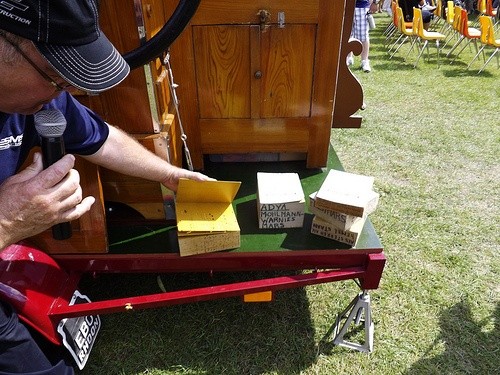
[68,0,347,203]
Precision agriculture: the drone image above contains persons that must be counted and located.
[0,0,216,375]
[346,0,379,73]
[378,0,500,32]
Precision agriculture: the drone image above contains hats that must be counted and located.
[0,0,130,93]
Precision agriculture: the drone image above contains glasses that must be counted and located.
[0,32,76,93]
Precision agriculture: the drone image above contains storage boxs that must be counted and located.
[252,168,381,247]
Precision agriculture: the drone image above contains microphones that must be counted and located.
[34,109,71,239]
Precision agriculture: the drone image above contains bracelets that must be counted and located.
[373,1,379,5]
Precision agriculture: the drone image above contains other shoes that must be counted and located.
[375,10,382,14]
[360,59,371,73]
[346,57,354,65]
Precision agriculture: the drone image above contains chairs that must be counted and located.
[382,0,500,75]
